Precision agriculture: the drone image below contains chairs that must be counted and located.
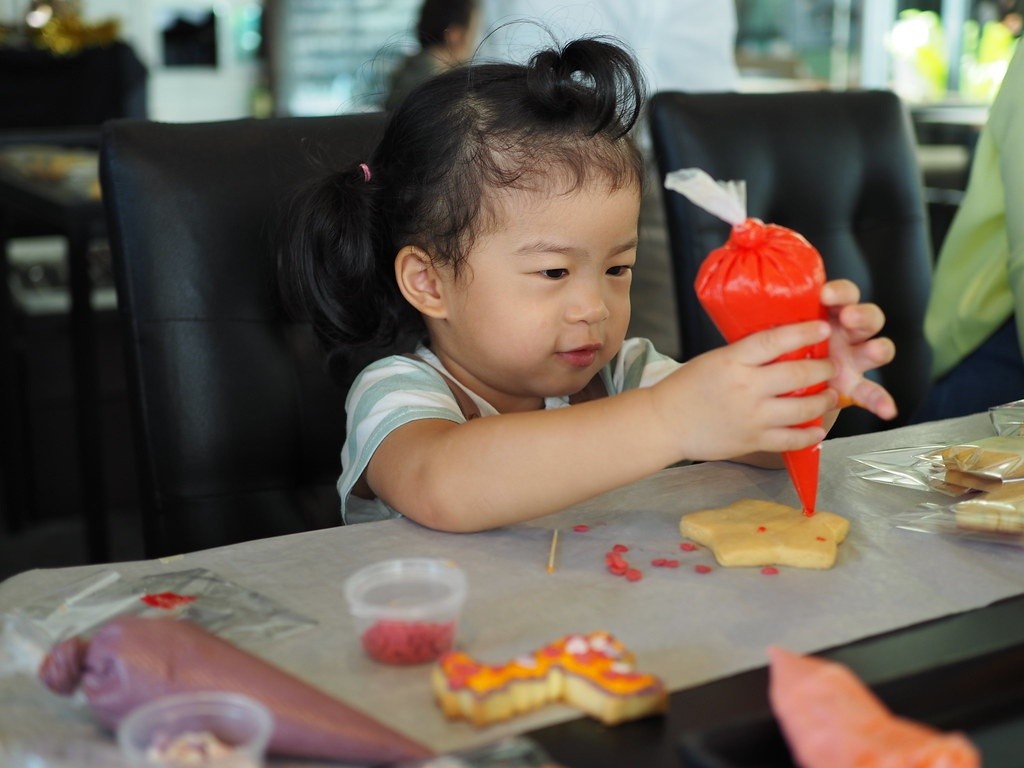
[648,89,933,439]
[97,110,388,557]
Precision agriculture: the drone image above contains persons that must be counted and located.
[274,34,899,534]
[377,1,485,116]
[919,34,1024,424]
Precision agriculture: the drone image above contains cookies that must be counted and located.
[679,499,849,568]
[431,629,664,725]
[942,426,1024,539]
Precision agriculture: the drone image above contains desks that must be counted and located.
[0,397,1024,766]
